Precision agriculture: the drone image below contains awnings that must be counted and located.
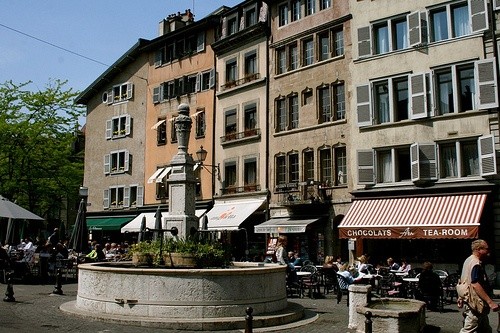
[199,197,266,232]
[72,215,137,231]
[254,218,319,233]
[337,190,491,239]
[121,209,207,234]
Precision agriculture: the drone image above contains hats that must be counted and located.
[357,255,368,263]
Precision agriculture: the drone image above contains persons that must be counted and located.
[456,240,500,333]
[0,227,132,272]
[322,251,412,297]
[272,234,303,272]
[412,262,442,312]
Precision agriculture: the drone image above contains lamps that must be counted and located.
[197,145,220,180]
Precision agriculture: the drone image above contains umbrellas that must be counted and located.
[67,198,89,257]
[152,205,164,241]
[137,215,148,243]
[0,194,45,244]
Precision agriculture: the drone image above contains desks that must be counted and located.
[316,266,324,281]
[395,272,408,282]
[403,276,446,303]
[390,270,401,276]
[57,258,75,280]
[293,266,301,275]
[296,272,312,297]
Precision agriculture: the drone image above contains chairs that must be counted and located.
[288,264,461,314]
[0,249,111,282]
